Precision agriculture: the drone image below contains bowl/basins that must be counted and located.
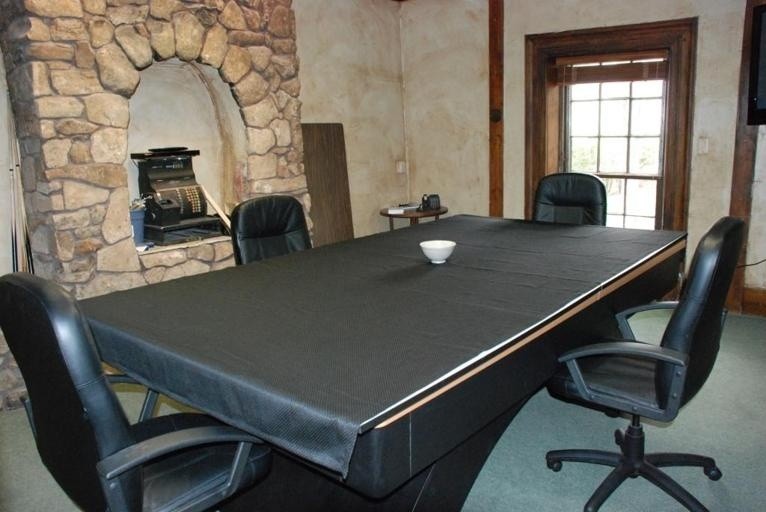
[419,240,456,264]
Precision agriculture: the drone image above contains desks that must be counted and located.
[379,204,448,230]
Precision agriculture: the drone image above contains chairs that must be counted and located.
[0,273,274,512]
[543,217,744,512]
[533,172,607,225]
[231,195,313,264]
